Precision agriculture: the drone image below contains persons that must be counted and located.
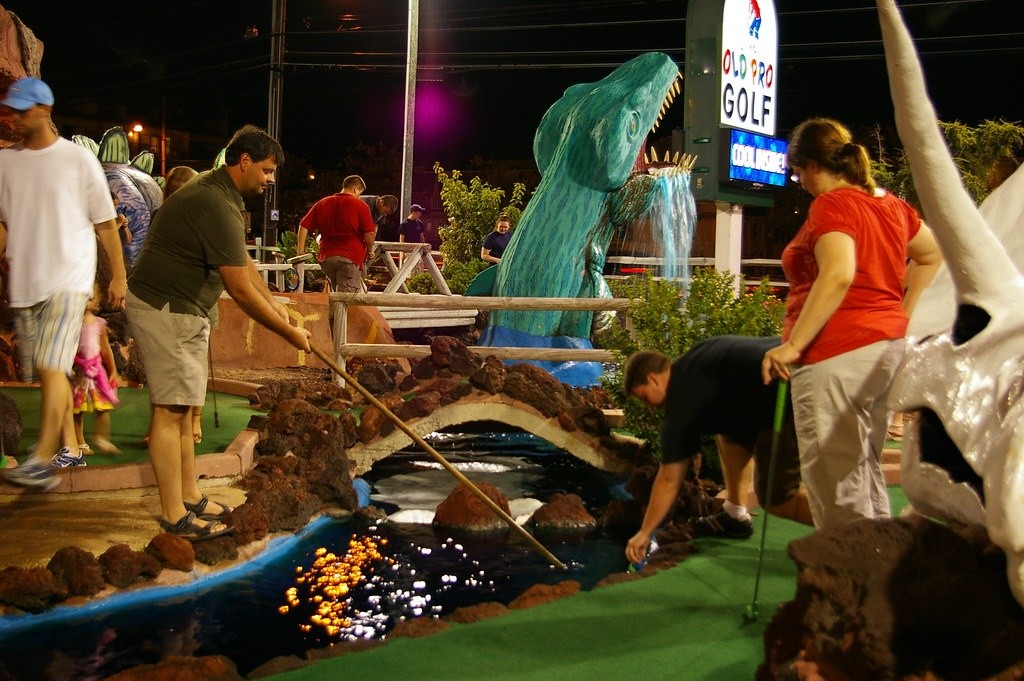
[481,215,513,265]
[399,205,425,245]
[0,77,131,485]
[762,120,943,537]
[140,165,224,448]
[624,335,803,562]
[70,284,123,455]
[297,175,376,295]
[111,193,133,246]
[360,195,399,215]
[124,127,312,542]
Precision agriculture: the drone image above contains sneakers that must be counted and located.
[1,460,62,486]
[688,510,754,539]
[49,446,87,469]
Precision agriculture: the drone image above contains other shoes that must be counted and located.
[92,436,123,454]
[78,444,95,456]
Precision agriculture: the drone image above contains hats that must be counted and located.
[1,77,55,110]
[409,204,426,213]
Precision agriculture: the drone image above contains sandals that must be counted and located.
[192,413,202,444]
[157,510,236,543]
[183,494,241,520]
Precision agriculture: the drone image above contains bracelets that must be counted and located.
[788,340,801,355]
